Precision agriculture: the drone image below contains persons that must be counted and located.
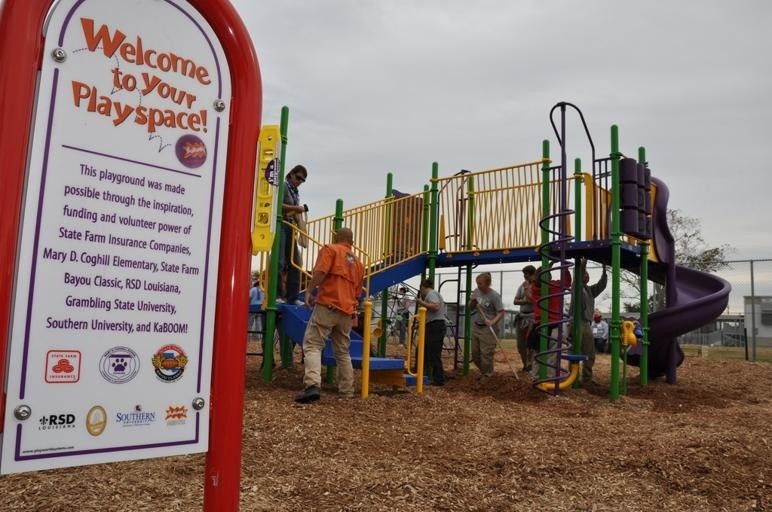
[415,278,447,386]
[275,165,308,305]
[294,227,366,403]
[397,287,410,346]
[513,259,609,389]
[467,272,505,381]
[247,281,264,342]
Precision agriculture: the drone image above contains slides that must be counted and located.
[619,265,731,378]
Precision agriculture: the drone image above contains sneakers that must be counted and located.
[291,299,306,305]
[276,298,285,304]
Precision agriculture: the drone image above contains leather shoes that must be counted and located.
[294,386,320,404]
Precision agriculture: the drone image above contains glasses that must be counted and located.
[295,173,305,183]
